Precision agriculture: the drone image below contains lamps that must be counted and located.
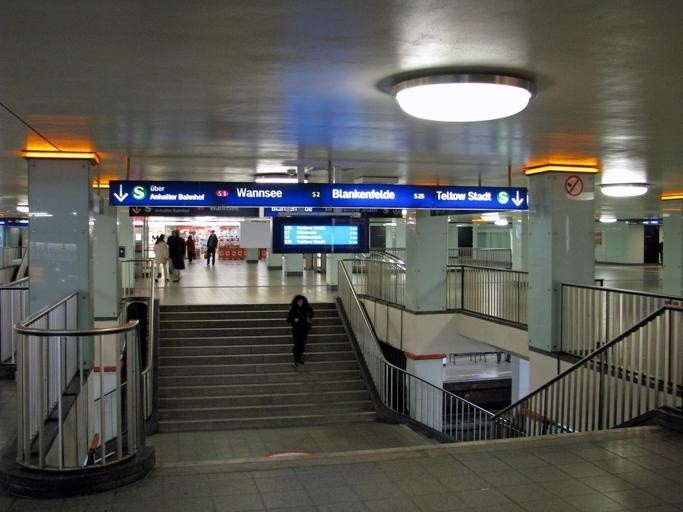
[600,183,648,198]
[254,175,309,184]
[391,73,538,122]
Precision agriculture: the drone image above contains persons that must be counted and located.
[184,235,195,264]
[205,229,217,266]
[153,230,186,282]
[286,294,315,369]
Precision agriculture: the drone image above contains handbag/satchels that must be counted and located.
[204,251,208,259]
